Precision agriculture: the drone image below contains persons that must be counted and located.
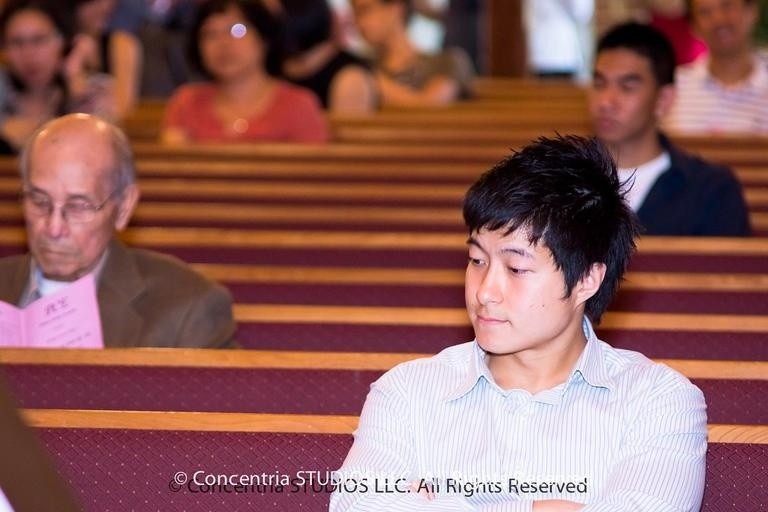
[328,130,709,512]
[0,371,81,512]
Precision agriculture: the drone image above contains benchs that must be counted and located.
[1,77,766,512]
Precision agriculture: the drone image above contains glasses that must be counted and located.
[22,190,119,218]
